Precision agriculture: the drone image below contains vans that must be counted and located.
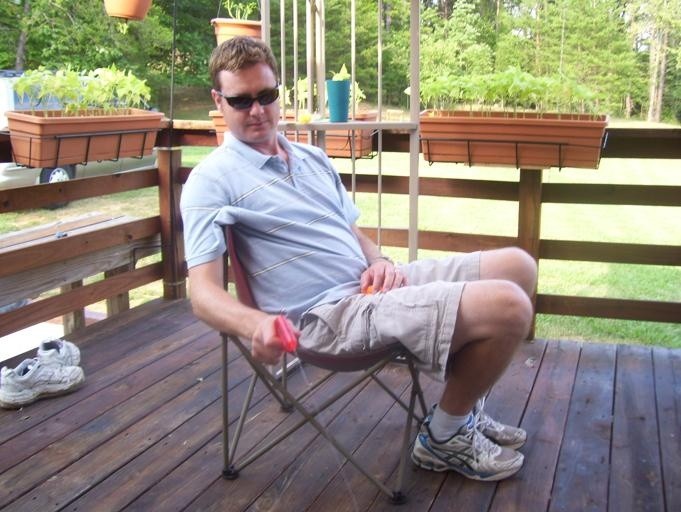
[1,70,168,210]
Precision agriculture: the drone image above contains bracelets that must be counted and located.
[366,254,395,267]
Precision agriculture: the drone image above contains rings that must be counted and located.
[394,267,403,273]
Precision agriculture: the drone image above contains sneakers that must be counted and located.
[0,357,84,410]
[38,336,82,366]
[410,398,526,482]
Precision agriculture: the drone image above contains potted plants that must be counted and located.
[405,72,610,170]
[211,1,263,46]
[4,67,170,169]
[208,64,378,158]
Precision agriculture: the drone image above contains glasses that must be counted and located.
[216,79,279,110]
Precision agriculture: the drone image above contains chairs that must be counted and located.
[221,223,428,504]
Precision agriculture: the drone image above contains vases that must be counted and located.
[105,0,152,22]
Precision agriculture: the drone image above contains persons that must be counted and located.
[179,32,541,483]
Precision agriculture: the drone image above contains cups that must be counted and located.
[327,80,350,123]
